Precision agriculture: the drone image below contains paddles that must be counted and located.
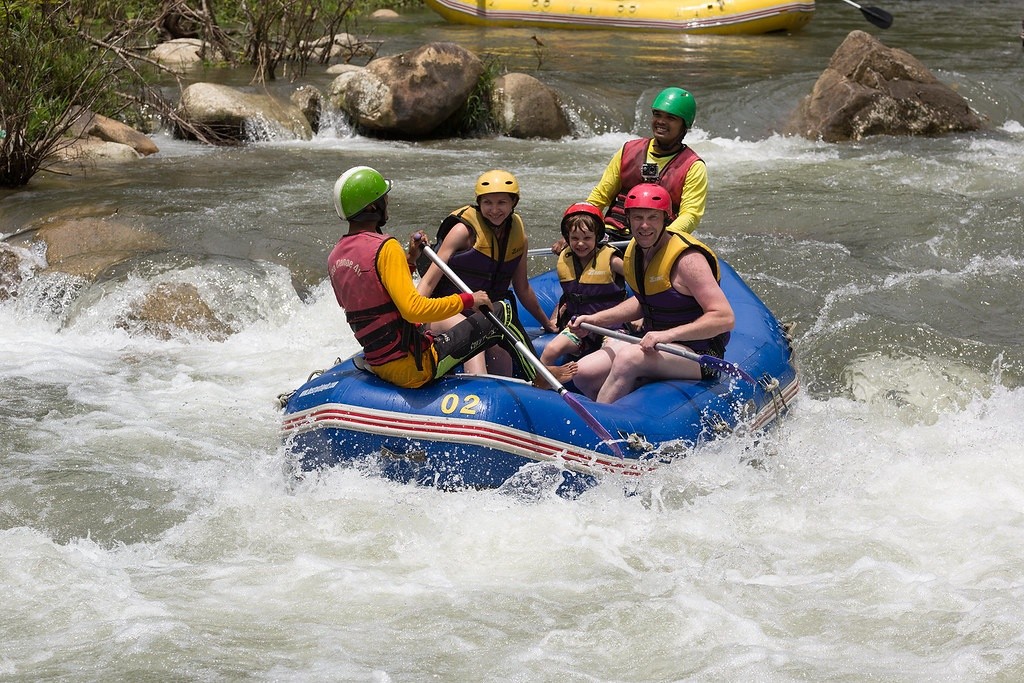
[571,315,757,386]
[413,232,627,461]
[526,240,630,257]
[842,0,892,30]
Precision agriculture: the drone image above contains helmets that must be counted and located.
[624,184,672,219]
[333,165,393,220]
[474,169,520,205]
[561,201,606,242]
[649,87,696,129]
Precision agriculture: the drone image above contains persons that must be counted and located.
[415,170,562,378]
[539,201,644,367]
[328,165,578,389]
[568,183,734,404]
[552,87,708,256]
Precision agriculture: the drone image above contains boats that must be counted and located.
[282,249,800,506]
[430,0,818,35]
